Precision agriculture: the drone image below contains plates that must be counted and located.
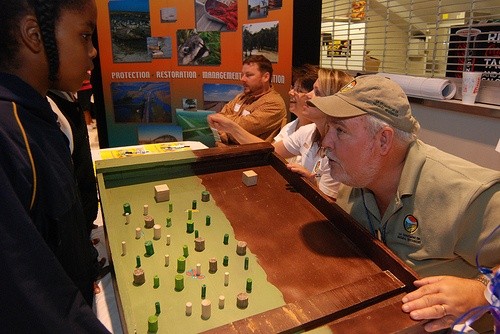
[456,28,481,37]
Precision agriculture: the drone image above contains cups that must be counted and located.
[462,72,482,104]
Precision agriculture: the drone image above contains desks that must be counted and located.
[95,141,453,333]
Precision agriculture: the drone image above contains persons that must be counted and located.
[0,0,112,334]
[307,74,500,333]
[217,55,286,144]
[207,65,356,204]
[47,70,112,281]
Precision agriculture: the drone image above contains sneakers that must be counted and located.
[93,225,98,229]
[94,281,100,294]
[97,257,112,280]
[92,238,100,245]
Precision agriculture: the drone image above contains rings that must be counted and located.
[441,305,447,318]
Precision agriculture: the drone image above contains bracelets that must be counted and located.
[475,277,490,288]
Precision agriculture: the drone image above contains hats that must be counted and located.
[306,74,416,133]
